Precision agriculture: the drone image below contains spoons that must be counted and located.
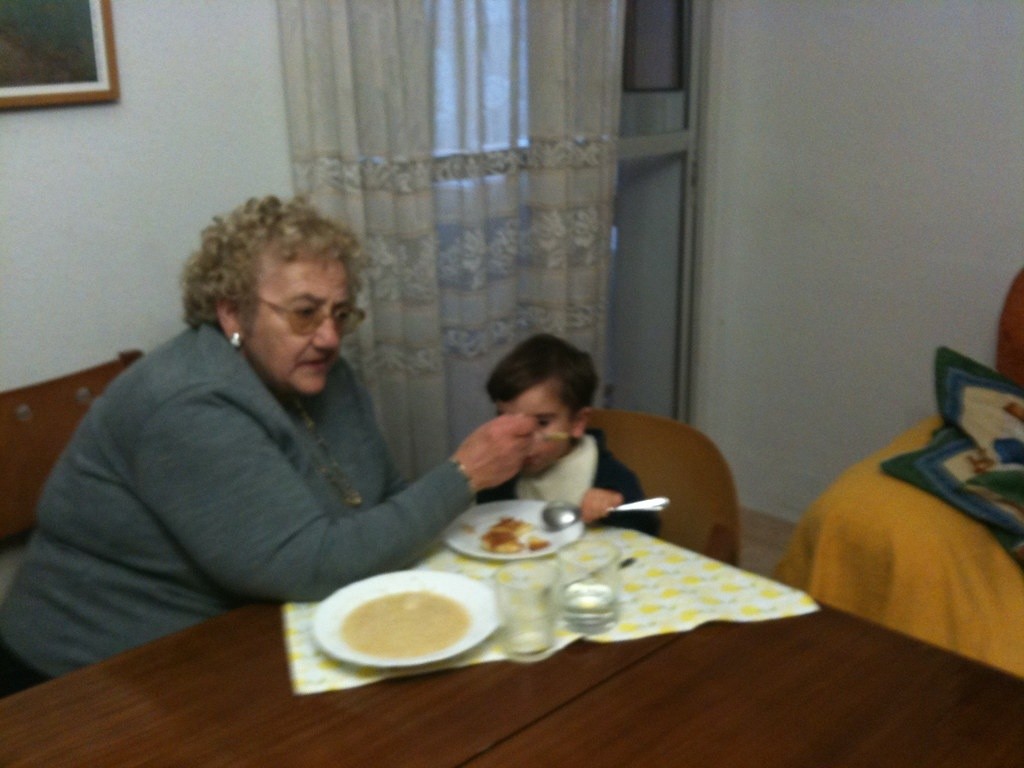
[542,496,671,530]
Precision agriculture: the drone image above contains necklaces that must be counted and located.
[292,393,364,507]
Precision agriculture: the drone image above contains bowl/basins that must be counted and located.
[309,569,499,668]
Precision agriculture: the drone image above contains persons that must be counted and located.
[471,333,664,537]
[1,195,537,702]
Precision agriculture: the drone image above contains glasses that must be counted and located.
[254,294,366,334]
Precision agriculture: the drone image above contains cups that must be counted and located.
[492,560,558,663]
[554,538,622,635]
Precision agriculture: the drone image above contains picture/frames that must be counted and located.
[1,0,118,108]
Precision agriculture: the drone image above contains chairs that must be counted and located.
[587,407,742,566]
[0,350,142,536]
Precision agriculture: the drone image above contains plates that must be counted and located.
[441,499,585,560]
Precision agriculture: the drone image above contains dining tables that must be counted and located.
[0,525,1024,768]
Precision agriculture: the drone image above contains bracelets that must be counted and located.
[449,457,478,503]
